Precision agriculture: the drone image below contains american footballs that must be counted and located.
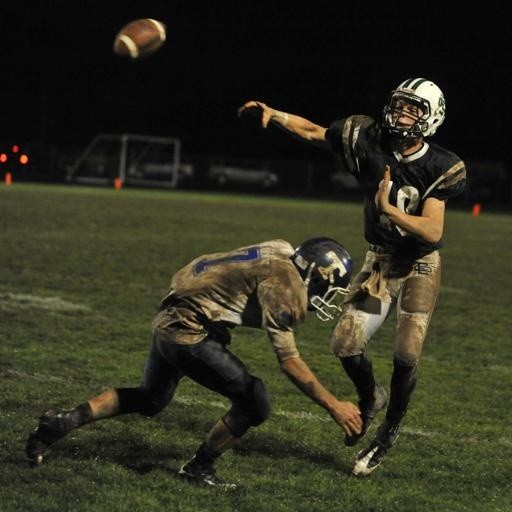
[114,18,166,61]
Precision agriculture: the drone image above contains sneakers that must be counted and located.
[352,425,398,475]
[345,386,386,448]
[179,455,237,491]
[26,410,68,458]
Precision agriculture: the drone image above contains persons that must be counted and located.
[24,237,364,487]
[237,76,468,474]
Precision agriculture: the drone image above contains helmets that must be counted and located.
[291,236,351,322]
[383,78,445,145]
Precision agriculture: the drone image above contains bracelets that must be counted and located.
[277,111,289,129]
